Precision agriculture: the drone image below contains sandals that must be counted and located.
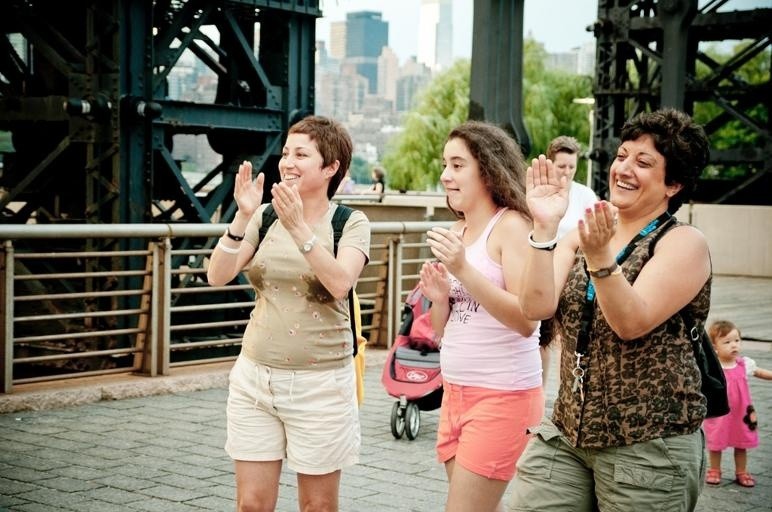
[706,468,721,485]
[736,473,755,487]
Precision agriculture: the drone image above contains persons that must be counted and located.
[698,320,772,490]
[339,168,358,195]
[363,168,386,202]
[541,135,600,399]
[204,113,373,511]
[519,105,717,510]
[417,119,555,512]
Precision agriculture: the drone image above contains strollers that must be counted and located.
[382,259,455,441]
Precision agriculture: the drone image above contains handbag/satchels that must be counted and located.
[689,325,731,420]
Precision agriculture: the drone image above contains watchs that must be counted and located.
[297,235,320,254]
[582,262,623,278]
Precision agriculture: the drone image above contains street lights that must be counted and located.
[573,97,595,189]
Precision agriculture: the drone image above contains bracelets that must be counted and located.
[527,230,557,251]
[215,237,240,255]
[224,225,246,242]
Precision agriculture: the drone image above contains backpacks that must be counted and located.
[258,201,367,405]
